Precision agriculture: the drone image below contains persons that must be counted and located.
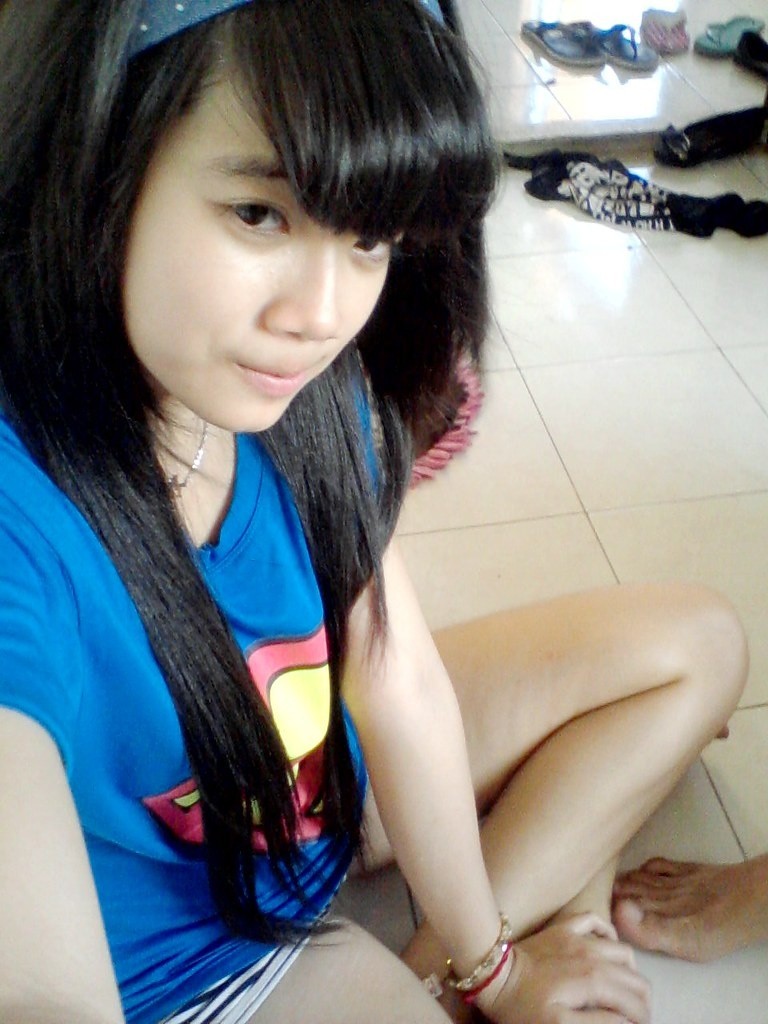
[0,0,750,1024]
[612,852,768,962]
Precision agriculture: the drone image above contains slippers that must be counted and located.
[521,19,605,67]
[566,22,658,70]
[642,10,689,55]
[693,15,765,59]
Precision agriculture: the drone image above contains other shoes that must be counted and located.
[654,107,768,169]
[736,31,768,78]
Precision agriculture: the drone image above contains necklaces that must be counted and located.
[166,419,210,491]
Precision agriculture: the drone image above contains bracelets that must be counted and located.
[443,911,515,1004]
